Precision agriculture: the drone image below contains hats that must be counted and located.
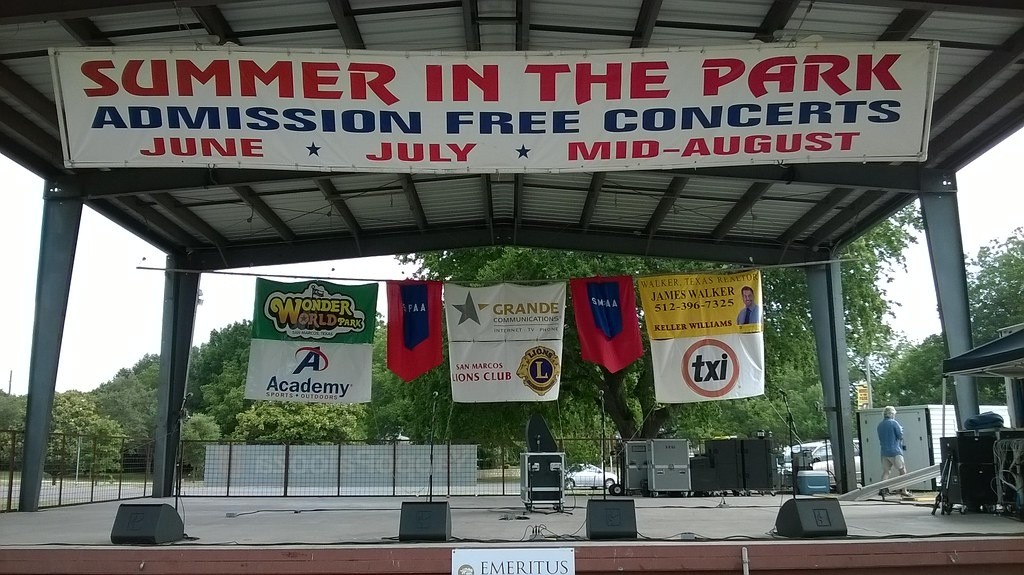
[883,406,896,414]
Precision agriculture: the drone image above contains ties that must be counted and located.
[744,308,751,324]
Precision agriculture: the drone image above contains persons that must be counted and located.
[877,406,914,497]
[736,286,759,325]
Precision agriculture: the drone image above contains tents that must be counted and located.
[942,328,1023,438]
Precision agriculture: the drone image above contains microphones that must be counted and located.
[779,389,787,396]
[599,390,604,395]
[433,392,439,398]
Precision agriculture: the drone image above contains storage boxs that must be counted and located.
[624,438,774,497]
[520,451,566,504]
[938,428,1024,521]
[797,470,830,496]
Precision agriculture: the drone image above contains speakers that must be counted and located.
[398,501,451,542]
[776,497,848,538]
[690,438,771,492]
[111,503,185,544]
[587,500,637,541]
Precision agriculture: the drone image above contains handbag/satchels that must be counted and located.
[900,438,906,451]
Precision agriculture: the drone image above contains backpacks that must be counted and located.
[964,411,1004,430]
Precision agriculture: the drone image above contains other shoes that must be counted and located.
[901,492,914,498]
[879,489,890,496]
